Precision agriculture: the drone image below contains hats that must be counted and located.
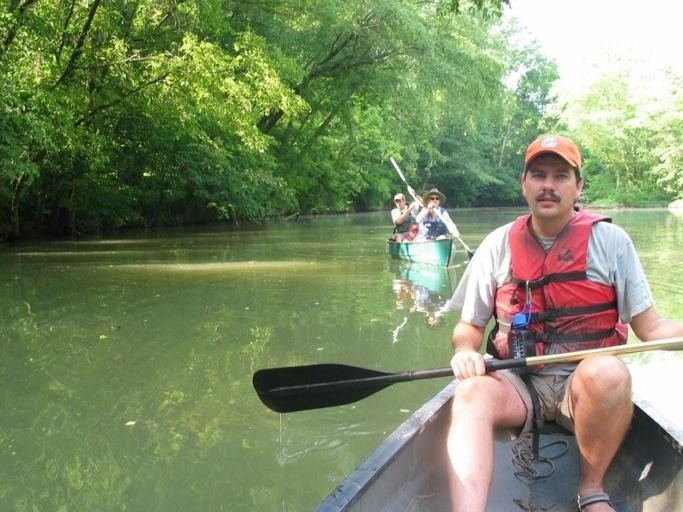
[394,193,405,200]
[423,187,446,207]
[408,223,419,231]
[524,135,581,171]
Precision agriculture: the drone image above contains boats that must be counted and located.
[314,324,683,512]
[314,351,683,512]
[387,237,456,266]
[384,220,456,269]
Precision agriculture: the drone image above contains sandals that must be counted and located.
[578,492,615,512]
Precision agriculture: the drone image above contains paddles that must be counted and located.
[432,207,475,260]
[252,336,683,414]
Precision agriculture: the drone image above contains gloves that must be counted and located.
[401,201,417,222]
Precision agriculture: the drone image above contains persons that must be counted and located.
[390,193,417,242]
[416,188,459,241]
[446,135,683,512]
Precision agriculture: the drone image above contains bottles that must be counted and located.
[508,312,537,377]
